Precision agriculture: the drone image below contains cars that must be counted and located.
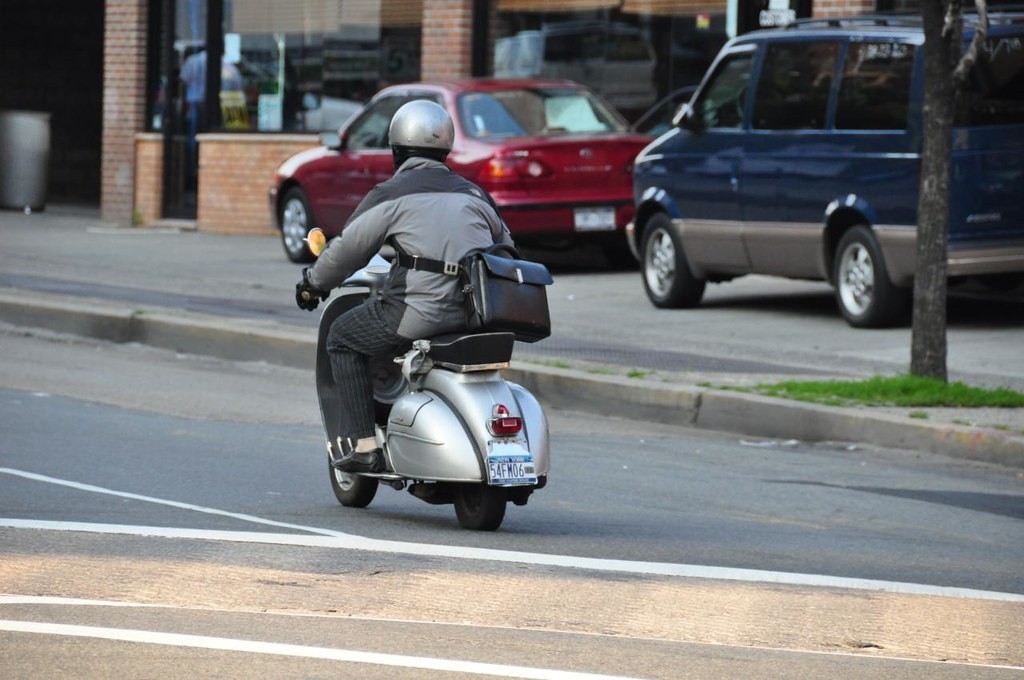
[265,75,659,264]
[151,21,733,133]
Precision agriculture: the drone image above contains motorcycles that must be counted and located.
[299,224,553,534]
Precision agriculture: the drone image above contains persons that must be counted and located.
[296,100,514,474]
[179,44,243,219]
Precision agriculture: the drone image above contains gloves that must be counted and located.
[296,268,329,311]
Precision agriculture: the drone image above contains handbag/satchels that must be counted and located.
[461,244,554,343]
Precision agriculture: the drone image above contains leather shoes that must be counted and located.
[331,447,384,472]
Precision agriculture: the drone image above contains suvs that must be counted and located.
[629,13,1024,330]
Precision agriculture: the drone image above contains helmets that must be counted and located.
[389,99,455,150]
